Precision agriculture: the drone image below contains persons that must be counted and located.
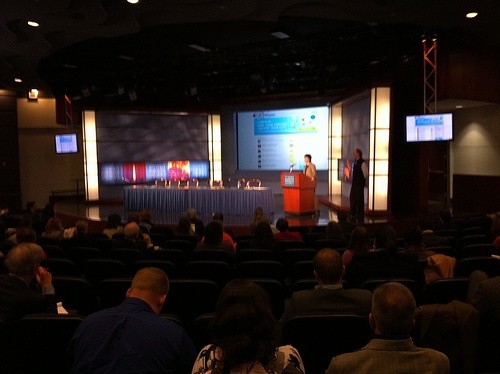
[324,282,449,374]
[192,279,306,374]
[0,242,58,315]
[303,155,320,213]
[277,247,375,328]
[468,270,500,320]
[0,202,448,287]
[348,148,369,222]
[66,267,200,374]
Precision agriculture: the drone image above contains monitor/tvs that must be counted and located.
[406,112,455,143]
[54,133,79,154]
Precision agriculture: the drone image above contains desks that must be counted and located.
[121,186,275,216]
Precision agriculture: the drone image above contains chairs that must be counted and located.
[0,216,500,374]
[154,177,262,187]
